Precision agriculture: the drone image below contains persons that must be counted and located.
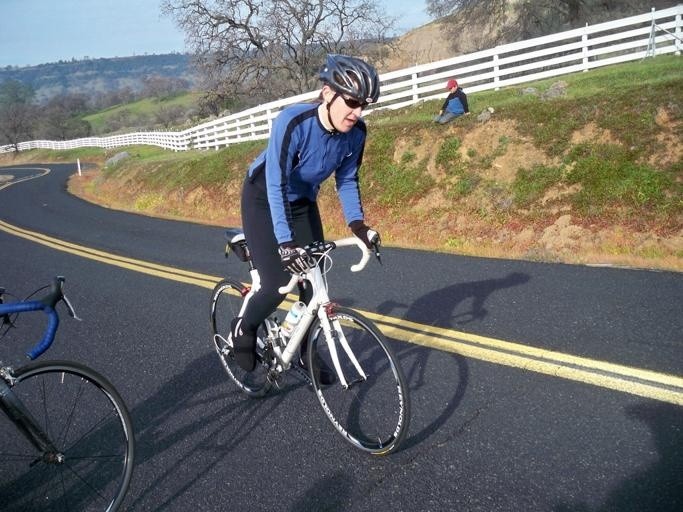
[434,79,471,125]
[230,54,380,385]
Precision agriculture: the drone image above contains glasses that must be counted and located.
[334,90,369,109]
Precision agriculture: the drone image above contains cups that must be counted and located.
[281,302,306,337]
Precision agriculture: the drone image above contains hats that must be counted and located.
[446,80,458,88]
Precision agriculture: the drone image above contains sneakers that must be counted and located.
[231,317,257,373]
[294,352,336,385]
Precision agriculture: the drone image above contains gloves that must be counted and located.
[349,220,381,253]
[278,242,317,276]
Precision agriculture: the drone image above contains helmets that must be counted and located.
[318,54,380,102]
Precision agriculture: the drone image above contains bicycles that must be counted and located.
[209,224,414,458]
[1,277,137,512]
